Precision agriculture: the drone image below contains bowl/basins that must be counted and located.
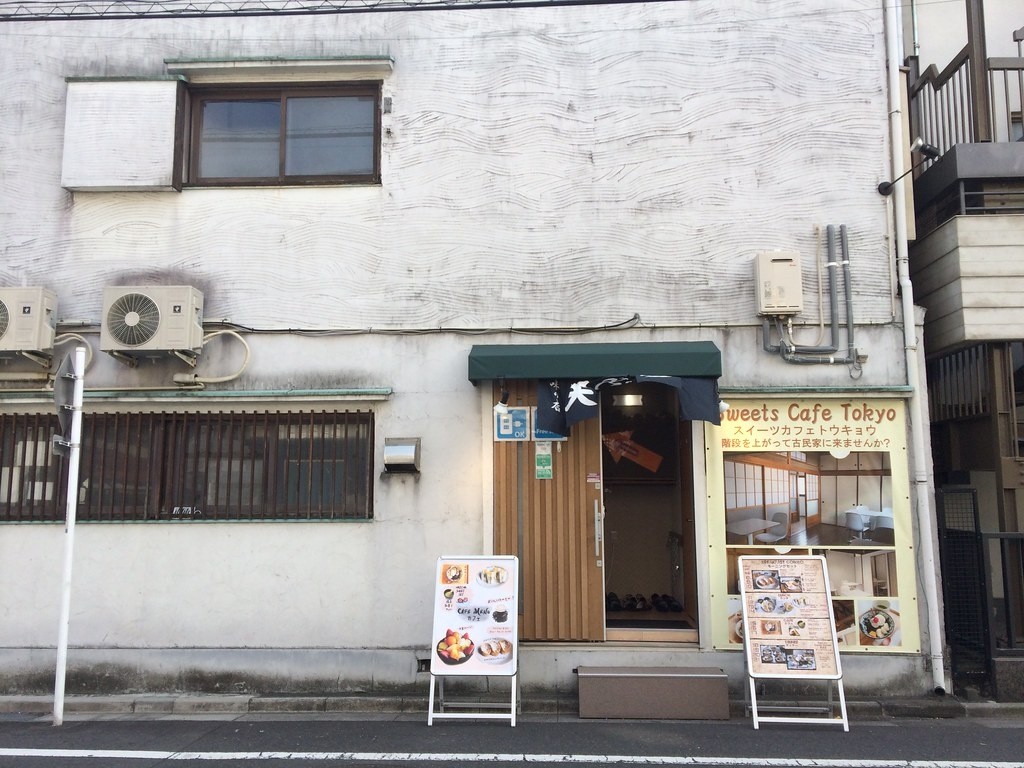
[444,589,454,598]
[436,636,475,665]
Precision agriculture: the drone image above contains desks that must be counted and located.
[857,510,894,526]
[725,518,780,545]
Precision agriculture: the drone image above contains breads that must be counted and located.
[480,640,512,656]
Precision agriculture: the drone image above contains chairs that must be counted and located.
[754,512,793,545]
[844,507,894,544]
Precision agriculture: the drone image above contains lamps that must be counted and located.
[877,137,940,196]
[718,399,730,413]
[492,374,512,415]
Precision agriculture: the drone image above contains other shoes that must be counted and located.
[662,594,683,612]
[606,592,621,610]
[624,595,637,609]
[652,593,669,613]
[637,594,647,610]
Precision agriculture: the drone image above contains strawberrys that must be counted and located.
[446,629,475,654]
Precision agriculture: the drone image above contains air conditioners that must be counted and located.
[0,285,59,352]
[98,284,204,356]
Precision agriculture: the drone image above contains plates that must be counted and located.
[475,638,513,664]
[859,609,894,639]
[761,600,775,612]
[756,575,776,588]
[446,565,463,582]
[477,565,509,587]
